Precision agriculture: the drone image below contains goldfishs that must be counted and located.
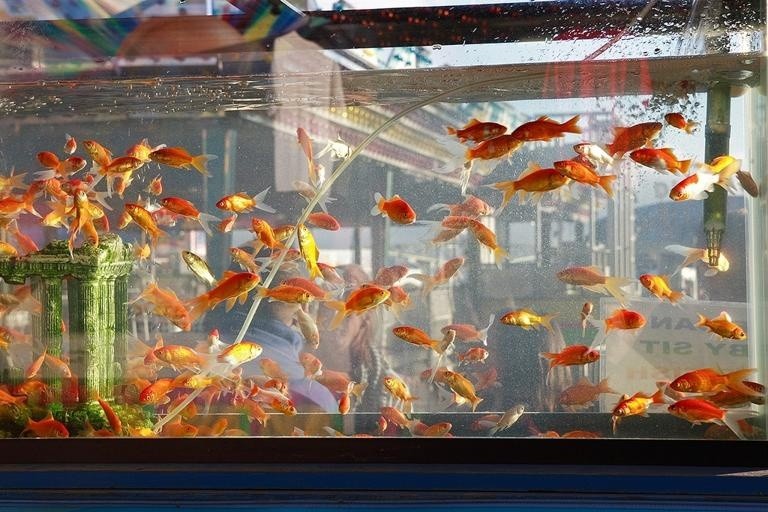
[0,112,767,441]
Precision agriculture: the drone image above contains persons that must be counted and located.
[134,233,342,413]
[471,308,600,412]
[302,260,413,414]
